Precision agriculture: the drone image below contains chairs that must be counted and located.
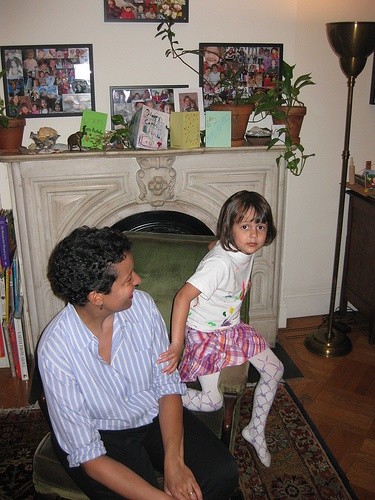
[28,231,252,500]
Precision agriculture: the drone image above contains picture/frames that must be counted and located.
[173,86,204,131]
[109,84,189,132]
[0,43,97,117]
[198,42,284,100]
[103,0,190,23]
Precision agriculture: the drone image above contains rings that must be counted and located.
[188,491,195,496]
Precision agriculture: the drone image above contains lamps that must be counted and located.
[305,22,375,357]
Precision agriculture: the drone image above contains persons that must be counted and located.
[6,49,91,116]
[111,115,131,150]
[185,99,198,111]
[108,0,185,18]
[142,89,156,109]
[152,92,163,110]
[168,92,174,111]
[133,93,142,100]
[181,95,191,111]
[161,90,169,113]
[203,46,279,107]
[156,189,284,467]
[134,102,141,112]
[37,225,246,500]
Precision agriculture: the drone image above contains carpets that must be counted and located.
[0,378,359,500]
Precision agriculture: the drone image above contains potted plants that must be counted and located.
[0,97,26,149]
[155,13,253,147]
[248,59,316,177]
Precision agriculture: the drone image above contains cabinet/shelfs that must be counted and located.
[339,190,375,347]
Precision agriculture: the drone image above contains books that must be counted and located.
[170,111,200,148]
[0,257,22,322]
[0,294,32,380]
[131,105,170,150]
[0,208,17,269]
[80,109,108,150]
[206,111,231,147]
